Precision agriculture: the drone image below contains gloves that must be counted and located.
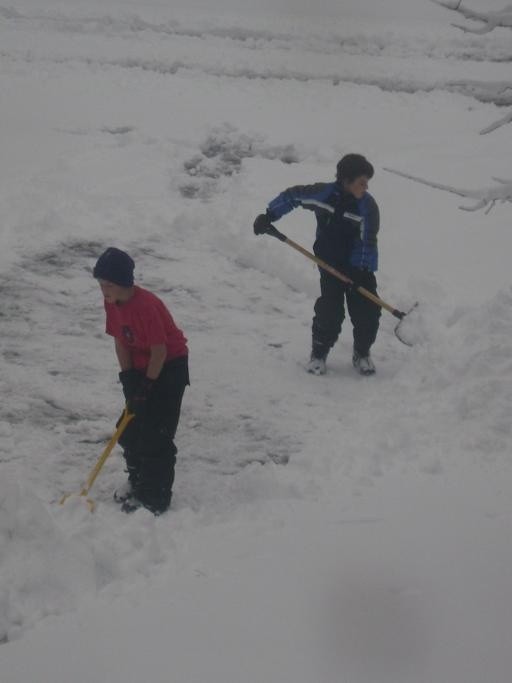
[254,213,270,235]
[349,268,364,294]
[117,371,156,415]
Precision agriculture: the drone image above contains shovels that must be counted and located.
[260,222,436,346]
[62,405,136,512]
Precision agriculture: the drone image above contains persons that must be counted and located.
[254,154,380,375]
[93,247,190,515]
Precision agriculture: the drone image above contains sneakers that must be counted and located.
[114,480,171,516]
[308,350,331,375]
[352,349,376,374]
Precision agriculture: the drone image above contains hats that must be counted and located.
[93,248,135,286]
